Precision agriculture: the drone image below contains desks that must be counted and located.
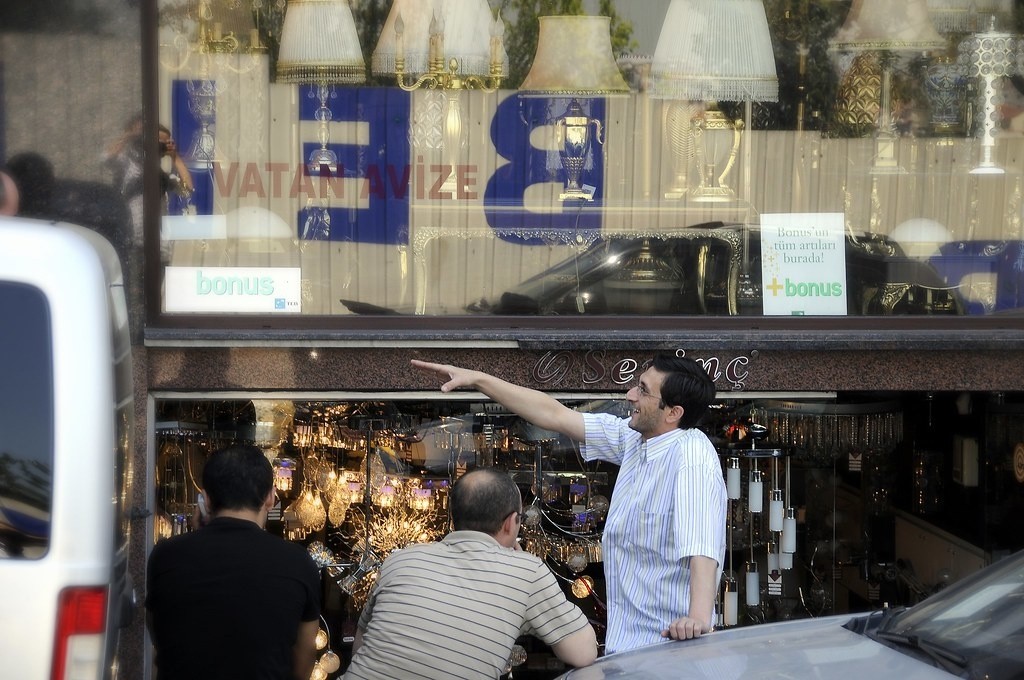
[395,198,751,317]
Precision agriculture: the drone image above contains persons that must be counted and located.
[411,354,727,656]
[146,444,320,680]
[337,466,597,680]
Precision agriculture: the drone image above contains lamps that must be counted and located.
[749,390,905,449]
[159,0,263,170]
[517,15,631,208]
[959,15,1024,174]
[393,6,506,194]
[155,399,611,680]
[914,451,941,515]
[275,0,367,173]
[920,0,1014,138]
[826,0,946,176]
[159,0,269,54]
[369,0,510,151]
[647,0,779,203]
[716,398,797,628]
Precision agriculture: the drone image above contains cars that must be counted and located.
[466,222,1024,316]
[543,544,1024,680]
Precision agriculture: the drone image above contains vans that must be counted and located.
[0,212,141,679]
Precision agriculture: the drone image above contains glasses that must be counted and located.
[503,511,528,524]
[633,381,667,403]
